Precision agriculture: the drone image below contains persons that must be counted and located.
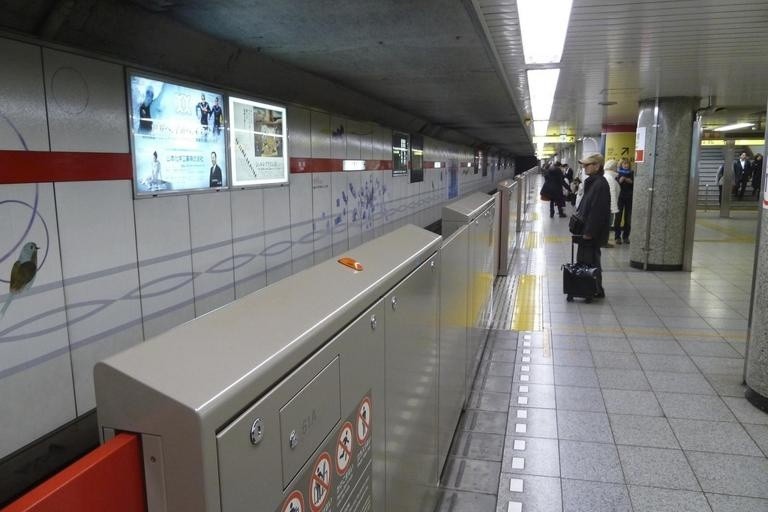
[209,152,223,188]
[138,90,152,134]
[569,153,611,299]
[196,94,211,136]
[715,152,764,206]
[141,152,162,188]
[209,97,224,136]
[540,153,634,248]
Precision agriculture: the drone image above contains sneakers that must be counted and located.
[600,239,630,248]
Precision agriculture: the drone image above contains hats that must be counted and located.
[577,153,603,165]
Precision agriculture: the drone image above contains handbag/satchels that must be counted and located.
[569,213,584,235]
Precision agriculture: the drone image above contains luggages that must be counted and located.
[560,236,599,303]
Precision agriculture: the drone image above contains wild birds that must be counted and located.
[0,242,42,320]
